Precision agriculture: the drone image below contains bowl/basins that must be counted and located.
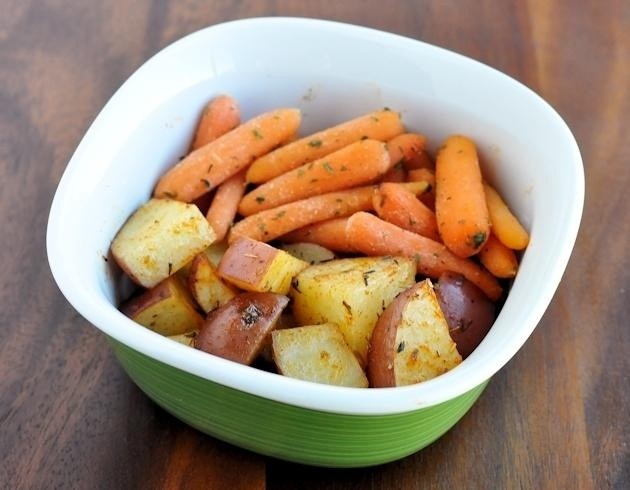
[43,14,588,468]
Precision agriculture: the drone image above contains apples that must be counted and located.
[110,198,497,388]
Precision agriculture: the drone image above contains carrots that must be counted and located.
[154,96,528,300]
